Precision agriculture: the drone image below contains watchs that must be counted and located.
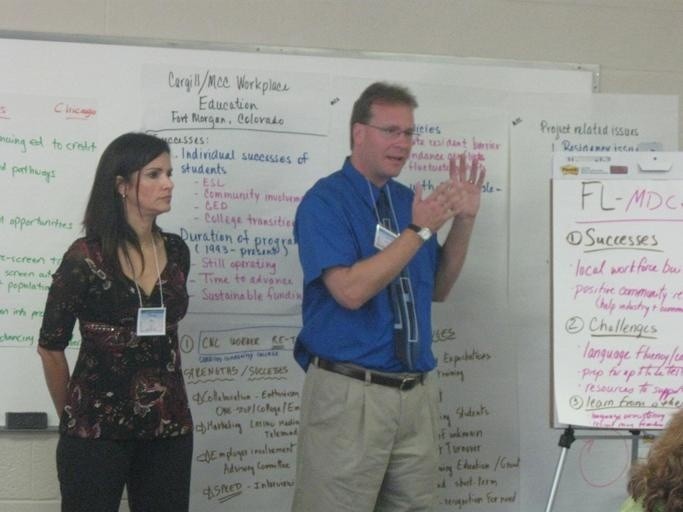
[408,224,433,242]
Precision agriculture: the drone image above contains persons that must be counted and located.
[37,133,193,512]
[290,81,485,512]
[618,407,683,512]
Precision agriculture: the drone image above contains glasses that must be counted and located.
[366,123,419,140]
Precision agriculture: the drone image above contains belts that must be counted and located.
[311,356,425,389]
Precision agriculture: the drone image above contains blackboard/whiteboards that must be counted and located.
[0,30,600,435]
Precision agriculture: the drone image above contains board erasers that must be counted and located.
[4,412,49,430]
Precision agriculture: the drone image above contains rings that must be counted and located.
[469,180,475,184]
